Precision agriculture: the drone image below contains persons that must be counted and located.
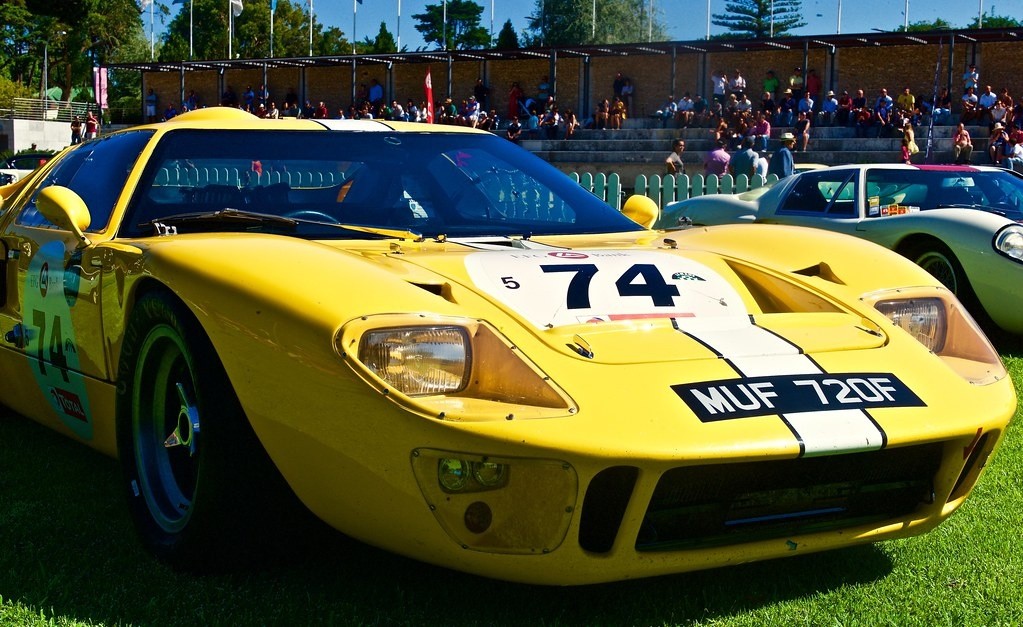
[145,89,157,123]
[0,157,19,187]
[161,72,633,144]
[70,115,82,145]
[85,111,97,141]
[31,143,37,151]
[651,65,1023,182]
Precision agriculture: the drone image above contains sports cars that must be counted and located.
[0,105,1018,596]
[649,164,1022,344]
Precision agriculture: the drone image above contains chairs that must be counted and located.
[902,182,975,211]
[792,187,829,212]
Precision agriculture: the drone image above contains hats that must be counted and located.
[479,111,487,115]
[512,116,518,119]
[784,89,792,94]
[469,96,475,99]
[259,104,264,107]
[780,133,796,141]
[840,91,848,95]
[969,64,976,68]
[304,100,310,104]
[445,99,452,104]
[545,110,550,113]
[712,98,719,102]
[992,122,1005,130]
[826,90,835,96]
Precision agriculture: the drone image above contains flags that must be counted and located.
[231,0,244,17]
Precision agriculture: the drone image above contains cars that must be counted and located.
[0,152,56,187]
